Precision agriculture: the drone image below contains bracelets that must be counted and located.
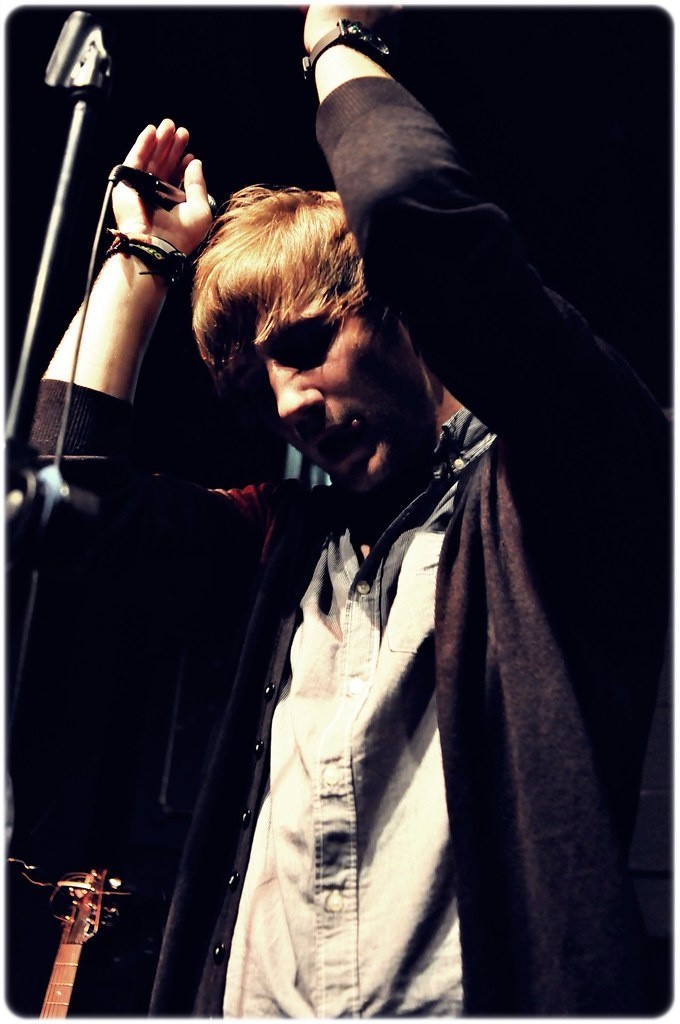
[106,229,187,292]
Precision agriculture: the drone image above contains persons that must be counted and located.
[6,4,673,1020]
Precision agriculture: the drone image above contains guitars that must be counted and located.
[30,861,136,1019]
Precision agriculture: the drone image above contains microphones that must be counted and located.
[109,165,217,217]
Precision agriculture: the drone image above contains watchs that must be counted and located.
[302,18,395,82]
